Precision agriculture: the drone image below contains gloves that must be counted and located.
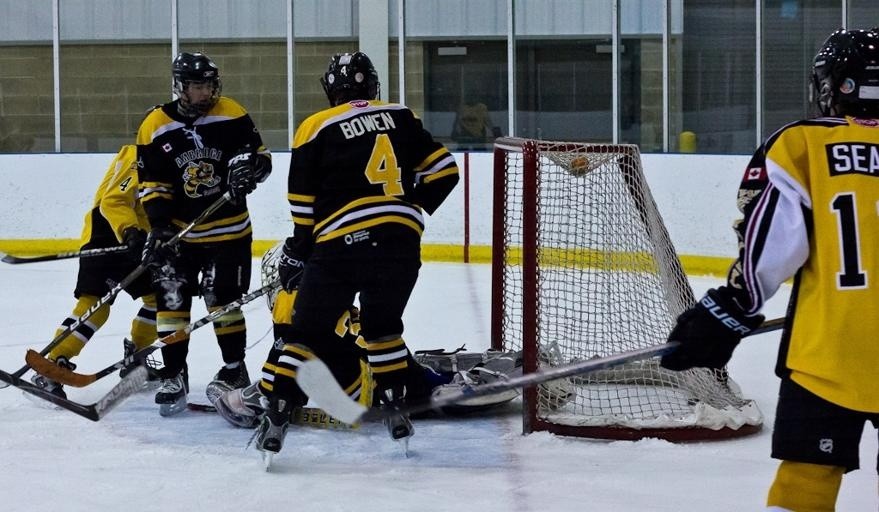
[277,236,307,285]
[145,236,166,267]
[667,286,763,373]
[124,230,138,257]
[226,150,258,197]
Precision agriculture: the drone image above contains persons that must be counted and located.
[205,235,574,427]
[136,51,273,417]
[258,51,460,470]
[660,30,879,511]
[31,103,160,400]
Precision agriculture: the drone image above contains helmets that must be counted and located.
[169,50,222,117]
[318,49,382,106]
[808,22,879,123]
[260,240,288,312]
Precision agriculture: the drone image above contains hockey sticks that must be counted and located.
[294,317,785,429]
[0,191,233,390]
[25,280,282,388]
[1,246,127,265]
[0,366,149,422]
